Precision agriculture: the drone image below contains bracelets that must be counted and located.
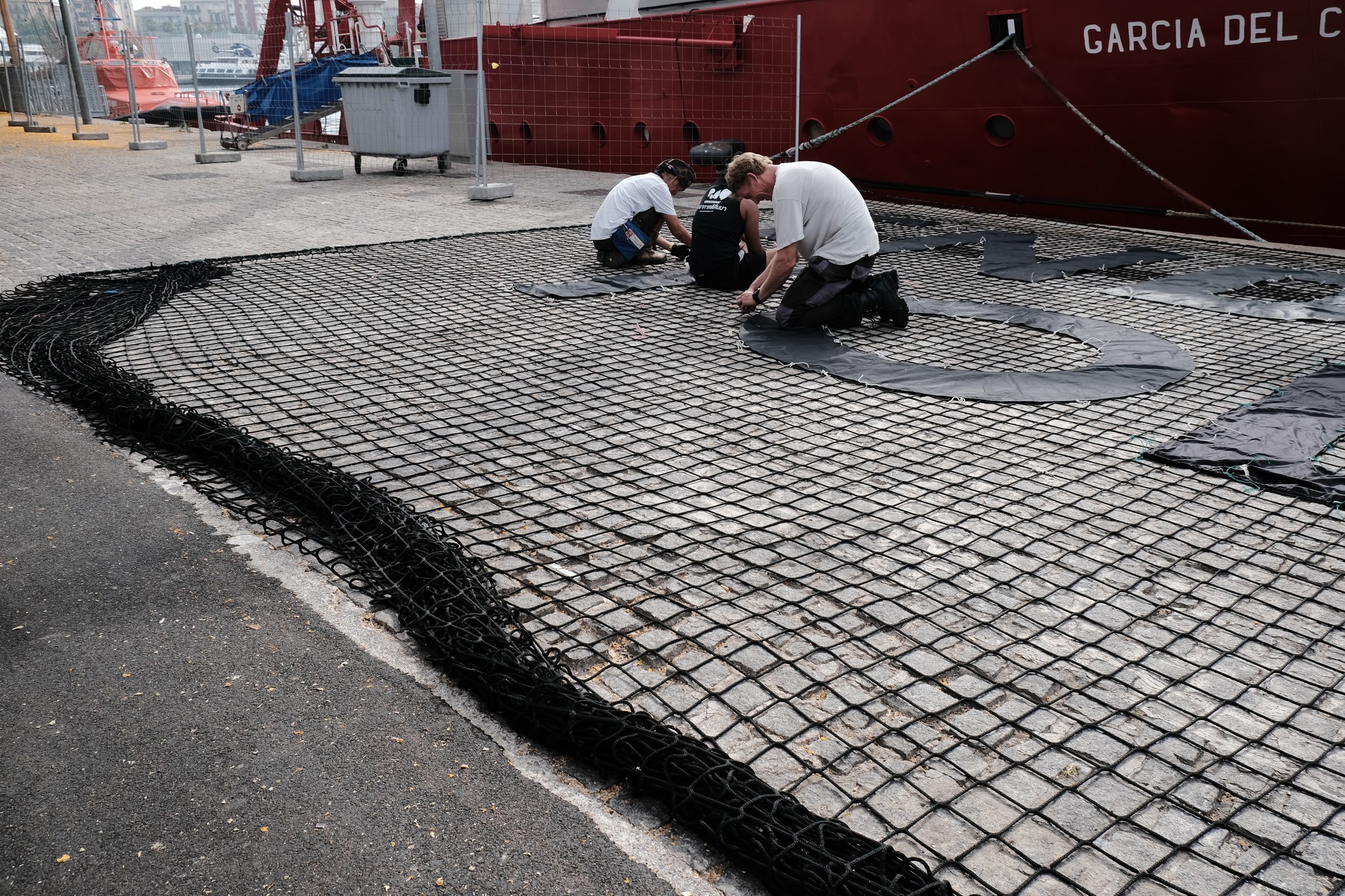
[668,242,674,251]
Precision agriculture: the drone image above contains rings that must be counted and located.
[738,299,740,303]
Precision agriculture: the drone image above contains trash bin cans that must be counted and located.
[334,67,452,172]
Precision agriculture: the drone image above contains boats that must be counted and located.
[0,0,234,131]
[190,41,299,88]
[201,1,1345,248]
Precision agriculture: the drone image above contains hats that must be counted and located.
[656,158,697,190]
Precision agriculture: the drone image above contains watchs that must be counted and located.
[752,288,767,303]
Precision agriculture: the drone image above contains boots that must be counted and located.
[859,266,909,328]
[636,228,667,265]
[596,251,624,267]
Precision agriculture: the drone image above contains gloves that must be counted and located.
[670,244,690,262]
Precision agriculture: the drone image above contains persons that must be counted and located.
[723,152,910,329]
[689,175,800,291]
[591,159,692,266]
